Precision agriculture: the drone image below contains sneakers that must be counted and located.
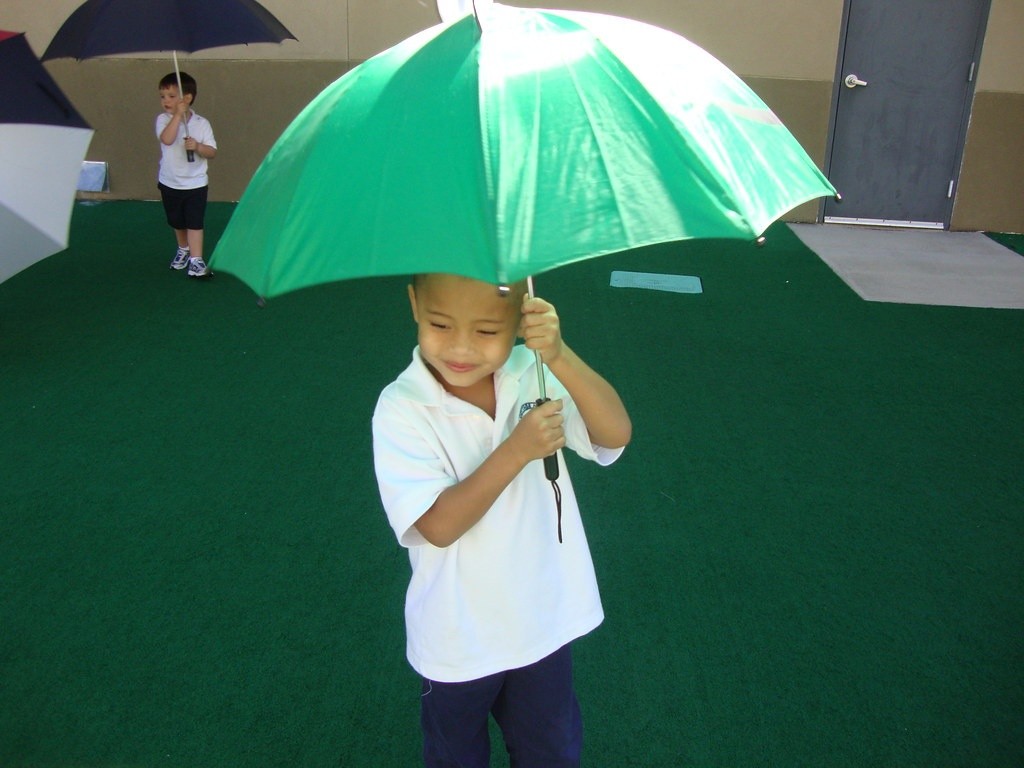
[171,248,190,270]
[188,260,214,278]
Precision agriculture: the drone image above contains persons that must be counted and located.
[367,269,637,767]
[153,71,218,277]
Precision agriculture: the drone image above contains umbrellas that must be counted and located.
[204,0,843,481]
[0,29,99,282]
[39,0,300,163]
